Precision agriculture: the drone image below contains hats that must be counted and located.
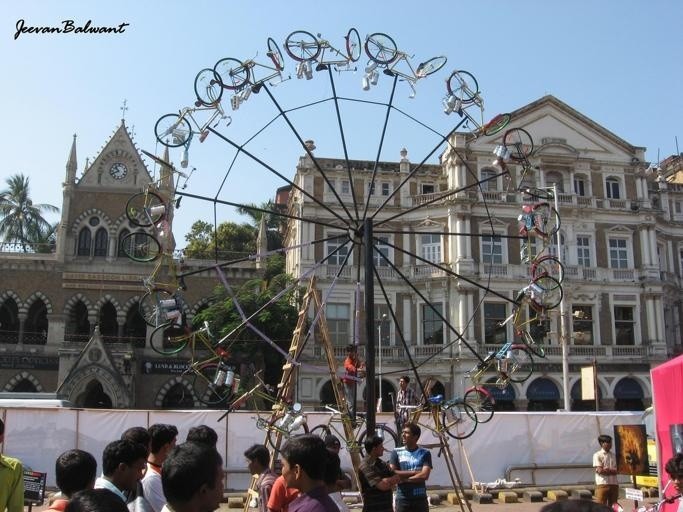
[325,435,340,447]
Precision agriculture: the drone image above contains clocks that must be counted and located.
[110,162,128,181]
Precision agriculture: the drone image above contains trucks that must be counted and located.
[627,402,658,492]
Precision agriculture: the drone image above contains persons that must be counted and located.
[395,375,415,436]
[592,435,619,507]
[0,419,25,511]
[664,453,683,512]
[343,344,366,424]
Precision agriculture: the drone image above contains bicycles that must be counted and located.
[120,26,565,464]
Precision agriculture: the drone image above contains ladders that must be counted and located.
[411,377,472,512]
[242,274,362,512]
